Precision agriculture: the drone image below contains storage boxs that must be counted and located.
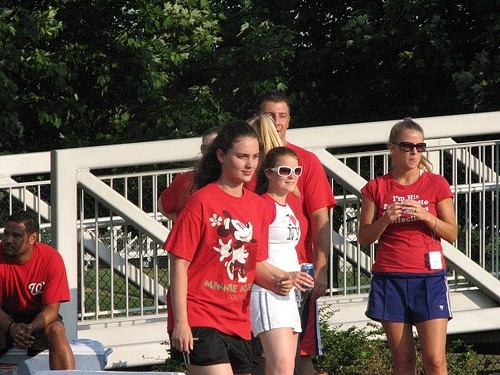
[0,338,113,375]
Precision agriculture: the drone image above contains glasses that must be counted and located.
[394,142,427,153]
[264,166,303,177]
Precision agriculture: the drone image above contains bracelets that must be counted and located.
[431,217,438,246]
[8,321,13,337]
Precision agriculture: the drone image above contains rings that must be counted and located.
[415,208,418,212]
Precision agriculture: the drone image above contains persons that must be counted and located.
[157,126,221,222]
[246,114,302,198]
[359,117,458,375]
[162,119,293,375]
[257,91,335,375]
[251,147,314,375]
[0,210,75,371]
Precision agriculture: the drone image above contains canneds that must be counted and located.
[301,263,315,290]
